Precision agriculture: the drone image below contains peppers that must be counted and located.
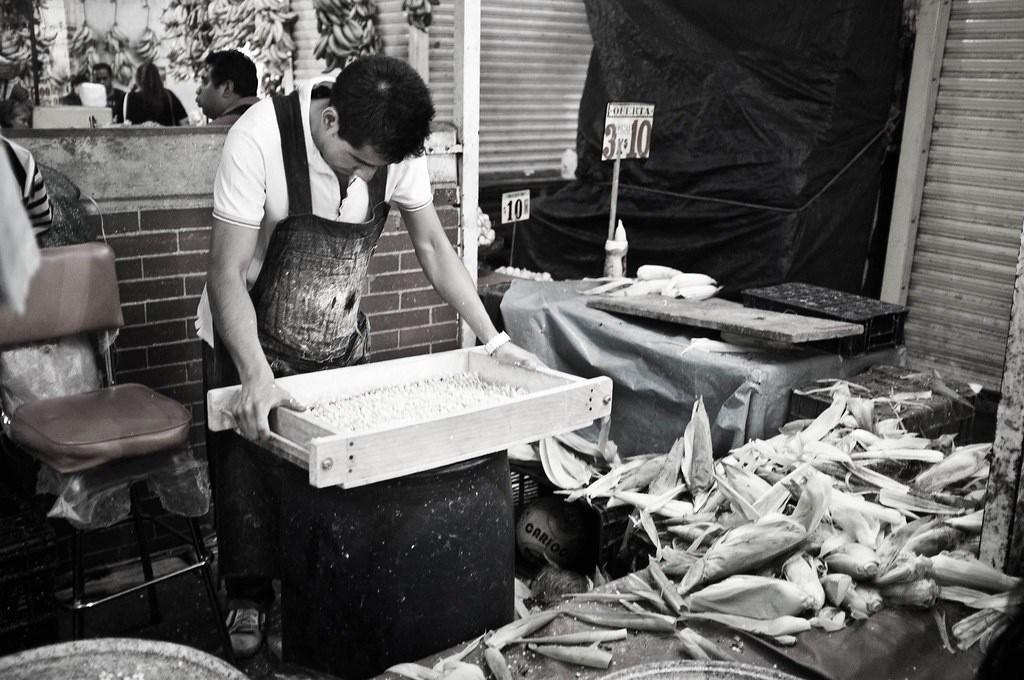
[0,0,447,99]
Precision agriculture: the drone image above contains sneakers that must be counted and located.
[226,608,268,656]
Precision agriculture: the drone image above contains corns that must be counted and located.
[567,398,1024,622]
[637,265,719,300]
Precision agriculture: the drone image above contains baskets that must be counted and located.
[505,458,633,578]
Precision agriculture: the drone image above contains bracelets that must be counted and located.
[484,331,512,356]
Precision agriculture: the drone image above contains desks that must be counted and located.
[374,461,1023,680]
[478,267,897,456]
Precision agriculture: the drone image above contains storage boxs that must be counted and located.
[788,364,976,448]
[506,461,629,581]
[207,346,614,490]
[742,280,910,354]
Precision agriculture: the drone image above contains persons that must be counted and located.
[0,135,53,228]
[0,100,29,128]
[62,76,90,106]
[195,56,540,657]
[116,62,189,126]
[194,49,262,127]
[92,63,126,123]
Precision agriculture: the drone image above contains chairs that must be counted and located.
[0,243,234,664]
